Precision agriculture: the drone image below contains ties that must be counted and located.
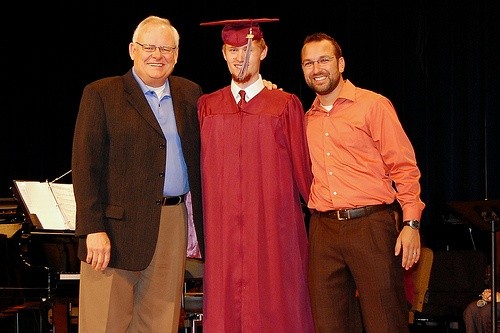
[237,90,247,110]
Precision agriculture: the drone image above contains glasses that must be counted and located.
[303,57,334,66]
[136,42,176,53]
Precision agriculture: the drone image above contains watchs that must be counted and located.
[402,219,421,230]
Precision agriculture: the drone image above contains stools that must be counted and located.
[0,301,43,333]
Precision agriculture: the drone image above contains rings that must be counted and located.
[413,253,416,255]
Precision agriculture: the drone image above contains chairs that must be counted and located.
[408,242,481,333]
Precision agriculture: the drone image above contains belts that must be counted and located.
[162,194,184,205]
[310,199,399,220]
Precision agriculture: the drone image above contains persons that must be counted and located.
[463,289,500,333]
[301,32,425,333]
[195,18,317,333]
[71,15,284,333]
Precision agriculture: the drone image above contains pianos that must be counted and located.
[0,177,80,333]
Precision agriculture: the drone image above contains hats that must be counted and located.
[200,19,279,80]
